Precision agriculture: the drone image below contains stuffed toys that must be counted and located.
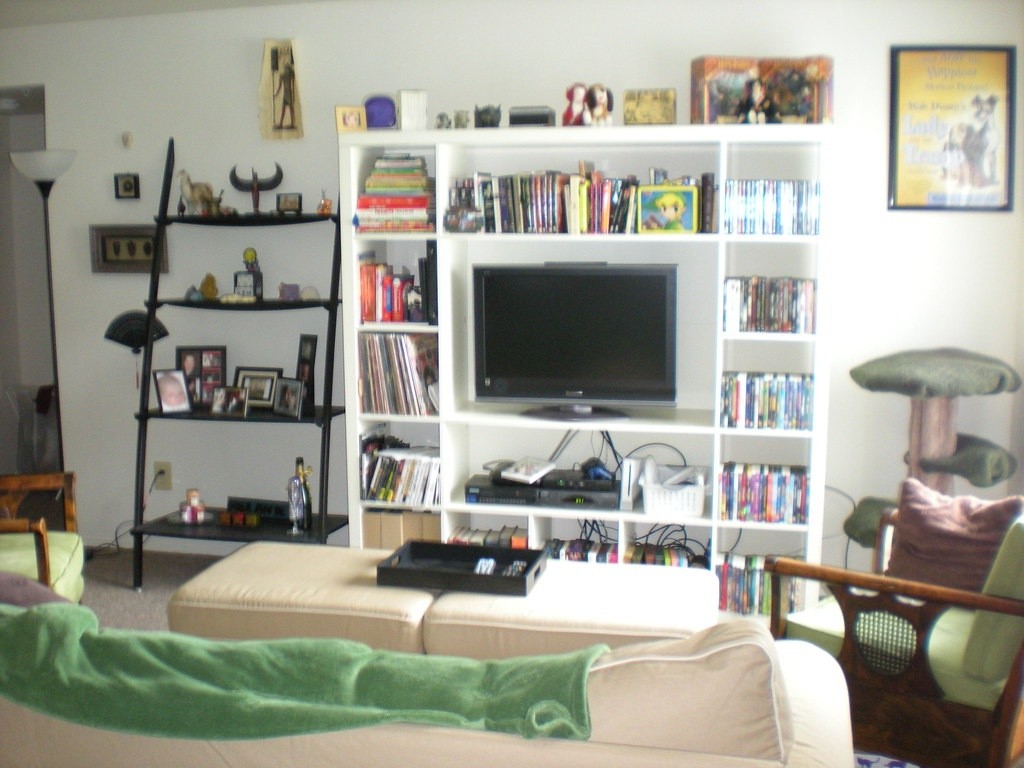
[562,84,613,126]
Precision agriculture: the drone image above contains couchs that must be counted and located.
[0,573,858,768]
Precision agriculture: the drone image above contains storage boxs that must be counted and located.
[638,463,706,515]
[376,540,547,599]
[757,56,833,122]
[692,55,758,121]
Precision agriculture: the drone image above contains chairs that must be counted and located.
[756,514,1024,768]
[0,469,87,603]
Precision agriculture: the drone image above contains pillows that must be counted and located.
[883,478,1024,593]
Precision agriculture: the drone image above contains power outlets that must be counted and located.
[154,462,171,490]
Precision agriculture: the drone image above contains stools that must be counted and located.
[169,540,723,653]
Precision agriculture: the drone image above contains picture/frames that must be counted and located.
[233,368,284,409]
[887,45,1014,212]
[89,224,169,275]
[637,188,697,232]
[209,384,250,417]
[297,334,319,418]
[176,345,226,409]
[274,377,304,420]
[153,370,192,413]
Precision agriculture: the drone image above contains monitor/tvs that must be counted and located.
[471,263,678,422]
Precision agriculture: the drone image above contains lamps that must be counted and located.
[6,149,93,565]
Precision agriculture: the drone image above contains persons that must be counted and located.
[182,353,201,377]
[745,78,776,124]
[157,376,188,412]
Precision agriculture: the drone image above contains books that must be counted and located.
[724,277,813,333]
[448,524,527,549]
[716,551,804,615]
[353,157,435,233]
[624,545,688,568]
[718,461,809,525]
[360,263,415,322]
[361,446,441,505]
[649,166,719,233]
[720,372,814,431]
[541,538,618,564]
[358,332,438,416]
[452,160,637,233]
[725,179,819,235]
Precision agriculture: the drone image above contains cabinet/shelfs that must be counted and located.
[340,122,832,619]
[127,141,343,594]
